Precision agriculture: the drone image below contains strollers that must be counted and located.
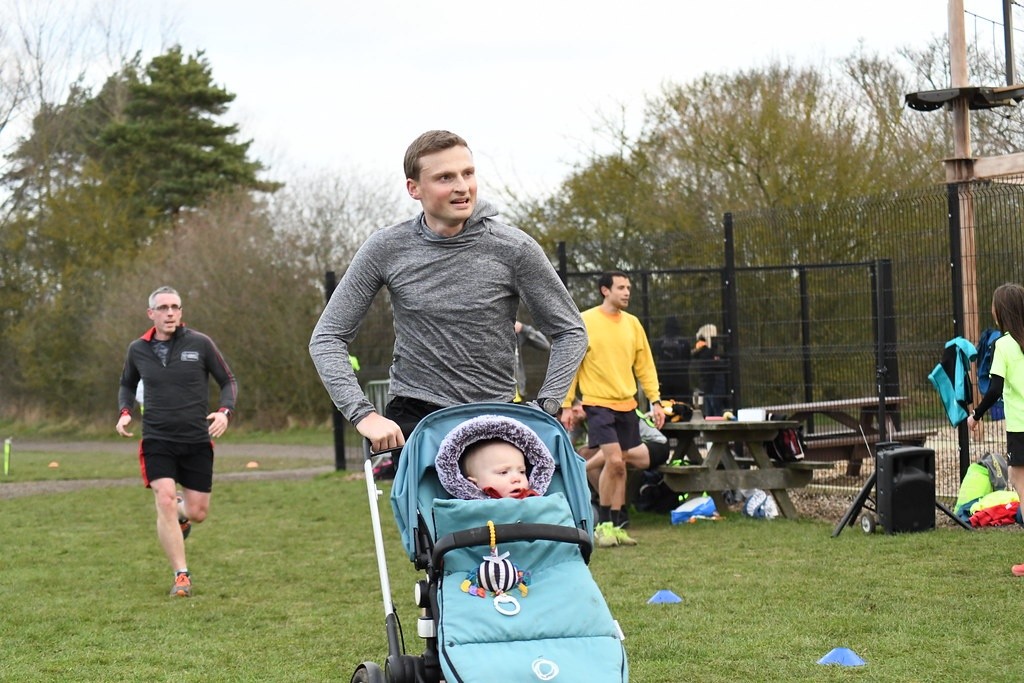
[349,398,631,683]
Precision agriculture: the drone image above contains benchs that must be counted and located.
[804,428,940,475]
[734,454,837,471]
[660,466,812,494]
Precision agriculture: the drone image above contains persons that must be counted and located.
[309,129,589,475]
[115,285,238,597]
[967,284,1023,576]
[436,415,619,682]
[562,269,665,549]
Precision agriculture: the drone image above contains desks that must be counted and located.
[756,394,912,434]
[658,420,813,519]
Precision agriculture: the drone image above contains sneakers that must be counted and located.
[594,521,617,547]
[168,570,192,599]
[615,526,638,545]
[176,490,191,540]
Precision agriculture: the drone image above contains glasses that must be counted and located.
[151,304,183,312]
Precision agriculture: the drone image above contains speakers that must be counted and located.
[876,446,936,534]
